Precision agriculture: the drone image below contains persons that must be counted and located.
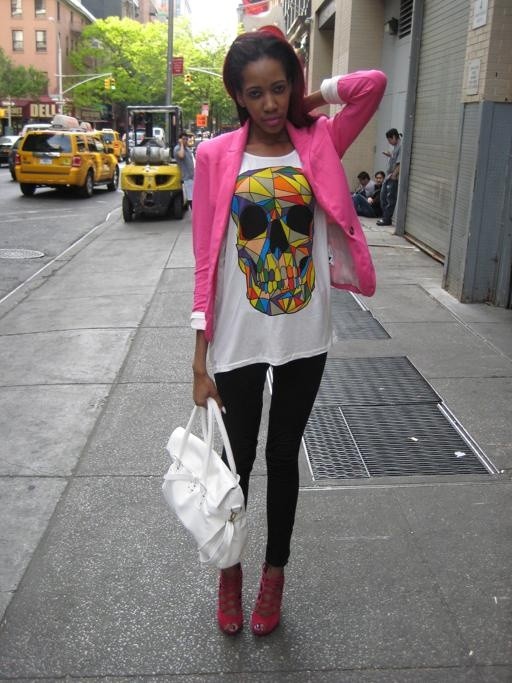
[190,24,388,637]
[375,128,403,227]
[212,133,217,138]
[353,171,376,199]
[351,171,386,219]
[208,135,210,140]
[173,132,194,210]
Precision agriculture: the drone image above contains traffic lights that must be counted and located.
[183,73,193,85]
[104,77,116,93]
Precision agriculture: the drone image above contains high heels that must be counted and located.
[217,563,244,635]
[249,563,285,636]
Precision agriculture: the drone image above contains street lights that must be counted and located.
[48,17,64,115]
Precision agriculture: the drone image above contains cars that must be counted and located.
[0,123,165,199]
[194,128,210,147]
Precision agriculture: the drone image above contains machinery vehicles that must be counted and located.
[120,104,195,222]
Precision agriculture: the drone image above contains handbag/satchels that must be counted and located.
[161,397,248,570]
